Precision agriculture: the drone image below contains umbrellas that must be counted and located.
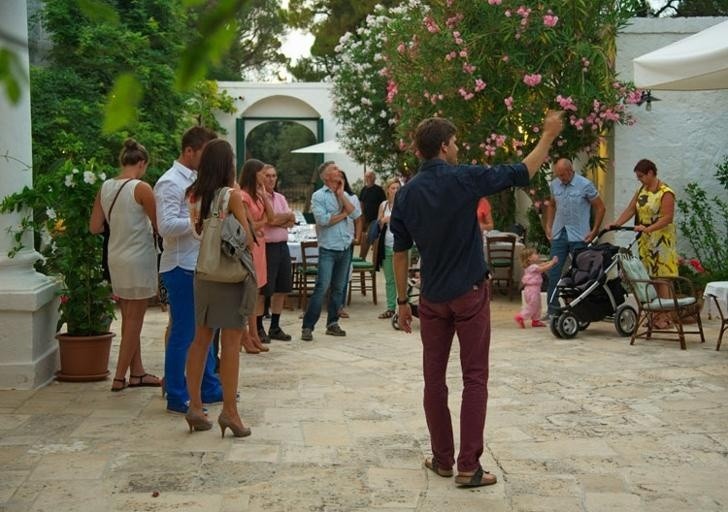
[631,19,728,91]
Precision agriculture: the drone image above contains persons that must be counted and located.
[515,249,559,330]
[387,109,566,487]
[302,161,362,341]
[86,138,164,395]
[238,159,273,354]
[544,156,607,321]
[185,139,252,438]
[605,156,680,330]
[256,164,295,343]
[159,273,220,400]
[359,171,388,250]
[327,171,363,318]
[152,127,241,416]
[378,178,412,319]
[477,197,493,235]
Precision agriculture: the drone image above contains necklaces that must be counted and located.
[646,178,659,192]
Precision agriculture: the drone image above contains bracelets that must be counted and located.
[397,296,409,305]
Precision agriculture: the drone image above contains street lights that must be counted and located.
[620,253,657,302]
[196,187,250,284]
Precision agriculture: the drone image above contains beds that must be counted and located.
[631,19,728,91]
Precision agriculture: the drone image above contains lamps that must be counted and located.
[619,257,705,350]
[290,235,517,312]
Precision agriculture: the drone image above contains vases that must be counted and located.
[0,157,123,337]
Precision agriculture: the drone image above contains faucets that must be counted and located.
[112,374,161,391]
[379,310,395,318]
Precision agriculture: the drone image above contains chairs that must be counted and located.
[167,402,207,413]
[516,316,544,327]
[302,329,312,340]
[326,325,345,336]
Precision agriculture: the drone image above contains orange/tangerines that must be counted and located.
[391,249,422,333]
[547,226,643,339]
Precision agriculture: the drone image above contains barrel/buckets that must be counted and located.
[455,467,497,485]
[425,456,453,477]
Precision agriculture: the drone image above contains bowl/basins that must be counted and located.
[646,178,659,192]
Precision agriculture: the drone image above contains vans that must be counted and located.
[185,409,212,432]
[218,414,250,437]
[240,329,291,354]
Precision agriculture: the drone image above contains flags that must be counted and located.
[54,332,116,383]
